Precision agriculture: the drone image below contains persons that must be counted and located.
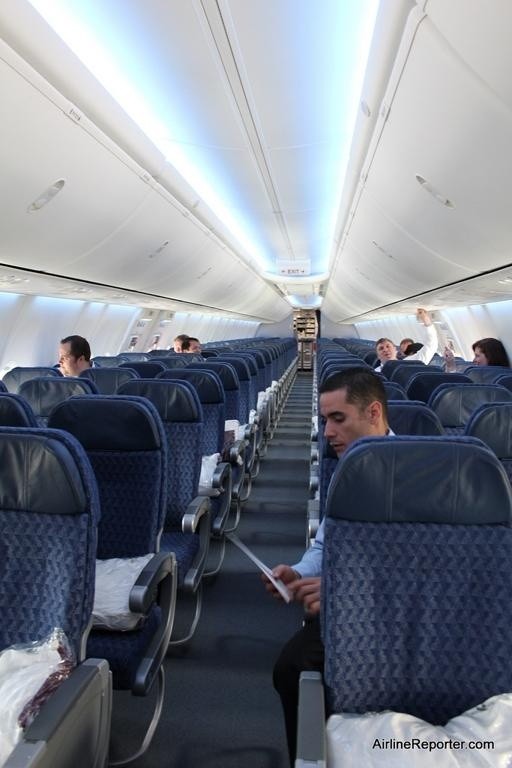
[261,364,400,768]
[403,341,425,357]
[398,337,416,355]
[180,337,202,354]
[471,337,510,366]
[55,333,100,379]
[372,307,439,372]
[172,333,190,353]
